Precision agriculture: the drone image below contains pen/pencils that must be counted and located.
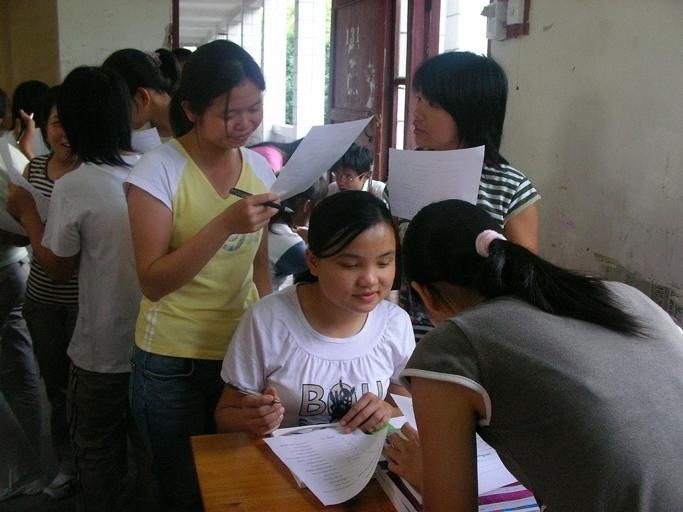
[16,111,34,144]
[369,171,372,188]
[229,186,295,215]
[226,381,281,404]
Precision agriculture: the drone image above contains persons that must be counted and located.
[327,141,386,203]
[245,137,305,177]
[381,198,683,511]
[9,79,51,158]
[6,85,92,500]
[99,48,178,144]
[0,89,57,498]
[266,185,318,291]
[214,190,418,435]
[121,40,284,512]
[390,51,543,342]
[153,46,193,80]
[4,66,145,512]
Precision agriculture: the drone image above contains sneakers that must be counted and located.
[0,471,43,500]
[44,464,84,502]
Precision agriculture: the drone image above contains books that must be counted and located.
[260,412,539,510]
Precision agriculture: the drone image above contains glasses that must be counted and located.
[333,169,368,183]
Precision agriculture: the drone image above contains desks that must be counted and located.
[189,431,395,512]
